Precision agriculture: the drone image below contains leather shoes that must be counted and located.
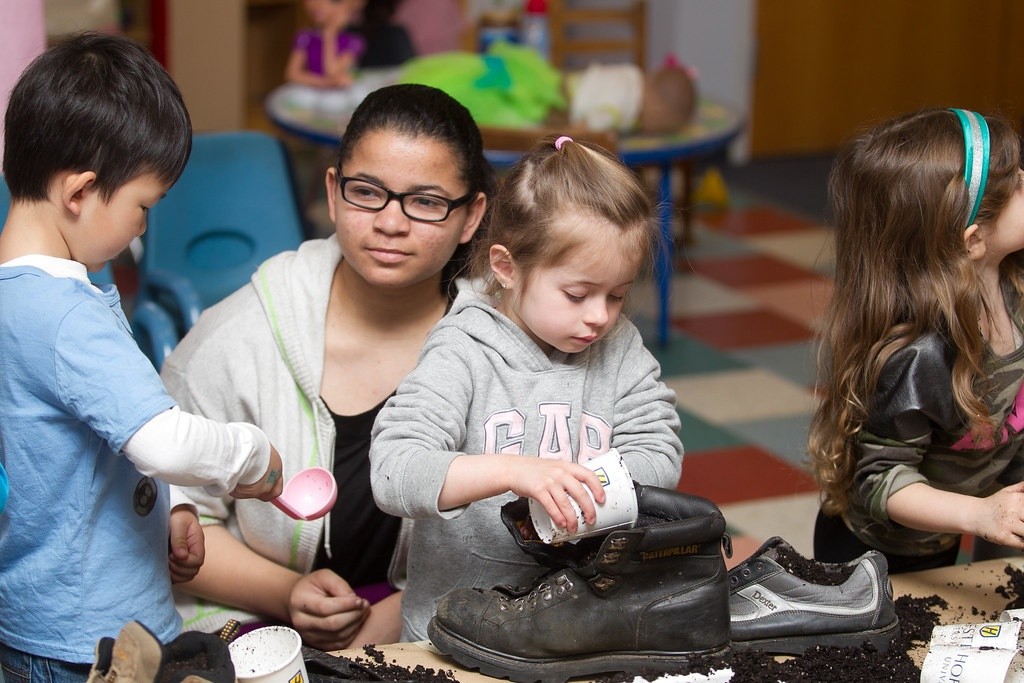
[427,478,732,683]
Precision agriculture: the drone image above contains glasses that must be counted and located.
[335,165,472,222]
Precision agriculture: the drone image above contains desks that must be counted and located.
[266,70,748,346]
[327,557,1024,683]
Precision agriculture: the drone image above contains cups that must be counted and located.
[527,447,639,545]
[920,609,1024,683]
[228,626,309,683]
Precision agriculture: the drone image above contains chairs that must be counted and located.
[0,173,202,375]
[138,132,302,314]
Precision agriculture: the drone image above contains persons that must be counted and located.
[284,0,364,89]
[367,135,683,645]
[804,109,1024,576]
[158,83,495,657]
[2,33,287,683]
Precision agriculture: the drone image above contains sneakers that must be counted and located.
[726,537,902,652]
[85,619,242,683]
